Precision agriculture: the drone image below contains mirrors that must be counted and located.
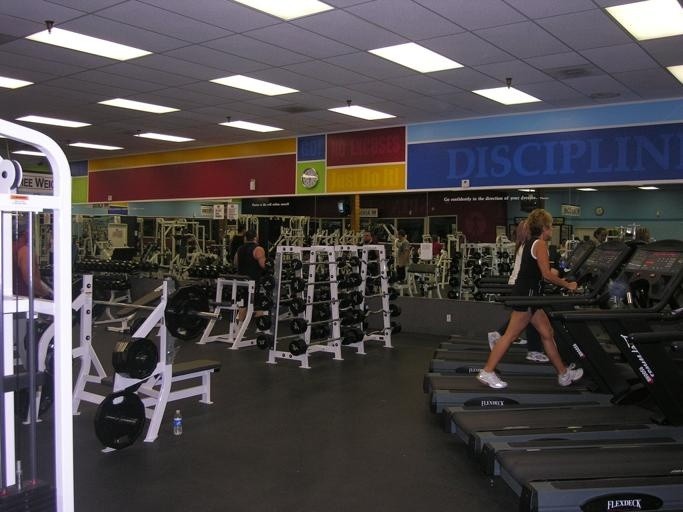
[72,183,681,304]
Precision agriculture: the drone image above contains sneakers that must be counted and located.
[526,352,549,362]
[558,368,583,386]
[512,337,528,344]
[476,369,508,389]
[488,331,501,351]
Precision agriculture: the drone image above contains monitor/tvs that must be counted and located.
[584,248,619,269]
[520,194,541,212]
[627,249,683,274]
[572,246,585,261]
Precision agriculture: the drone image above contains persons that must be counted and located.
[590,226,608,246]
[358,231,378,274]
[395,229,412,282]
[476,209,584,390]
[228,226,270,280]
[13,224,54,300]
[432,234,443,256]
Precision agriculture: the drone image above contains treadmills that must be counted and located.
[424,240,682,510]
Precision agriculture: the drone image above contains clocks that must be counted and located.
[299,167,319,189]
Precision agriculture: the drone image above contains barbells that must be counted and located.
[447,252,510,301]
[16,370,146,449]
[23,322,158,379]
[71,278,220,340]
[257,257,401,354]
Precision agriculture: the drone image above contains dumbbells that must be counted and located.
[186,265,236,302]
[39,257,158,289]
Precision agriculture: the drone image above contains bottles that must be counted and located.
[172,409,183,437]
